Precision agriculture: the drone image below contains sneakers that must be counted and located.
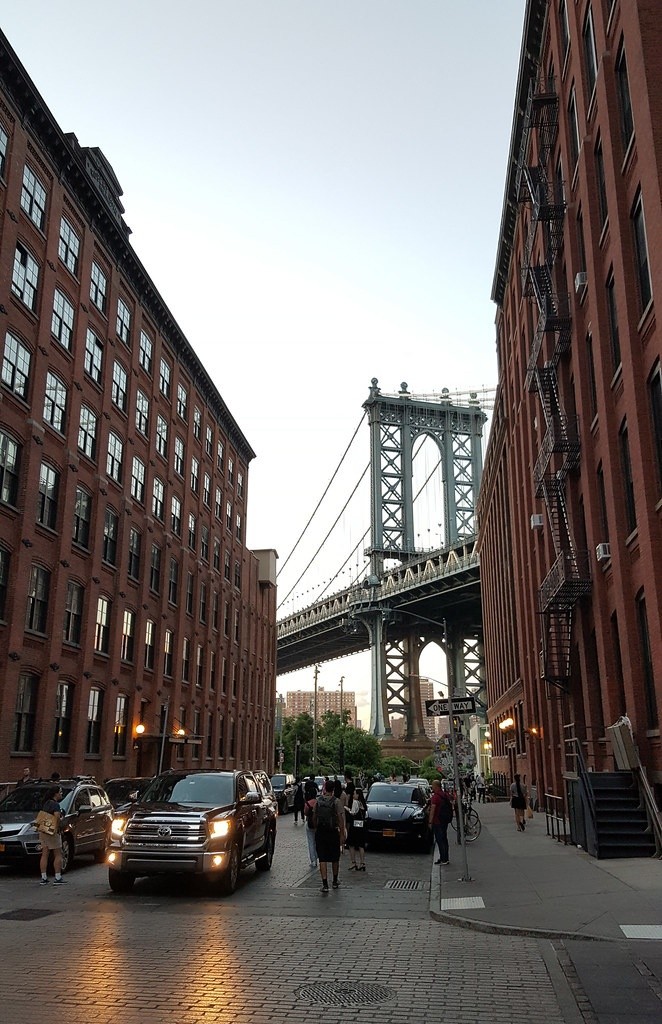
[40,877,50,885]
[53,877,69,885]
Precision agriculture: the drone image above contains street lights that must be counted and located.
[313,665,323,775]
[338,676,345,775]
[296,738,300,783]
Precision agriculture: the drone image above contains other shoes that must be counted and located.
[523,819,526,824]
[519,822,525,831]
[320,886,328,891]
[311,860,317,867]
[434,859,450,865]
[294,821,298,825]
[332,880,341,888]
[516,829,521,832]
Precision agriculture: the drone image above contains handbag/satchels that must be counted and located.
[349,807,367,833]
[32,801,60,836]
[526,800,533,818]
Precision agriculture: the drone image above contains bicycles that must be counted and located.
[452,799,481,842]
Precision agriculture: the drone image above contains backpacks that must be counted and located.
[316,794,340,829]
[436,794,453,824]
[294,784,304,801]
[305,802,315,829]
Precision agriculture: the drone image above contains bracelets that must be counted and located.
[429,823,431,824]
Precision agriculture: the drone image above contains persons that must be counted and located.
[16,767,34,789]
[313,781,345,891]
[322,774,349,805]
[343,774,355,809]
[304,774,320,801]
[344,789,368,871]
[510,774,528,831]
[290,777,305,825]
[40,786,69,885]
[304,788,320,866]
[460,772,486,804]
[428,780,450,865]
[51,772,59,782]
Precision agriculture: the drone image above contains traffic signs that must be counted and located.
[425,696,476,717]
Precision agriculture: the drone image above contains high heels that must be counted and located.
[357,863,366,871]
[348,862,358,870]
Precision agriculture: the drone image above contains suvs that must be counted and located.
[403,779,434,800]
[363,782,434,854]
[0,776,115,876]
[267,773,347,815]
[105,767,278,898]
[102,777,155,818]
